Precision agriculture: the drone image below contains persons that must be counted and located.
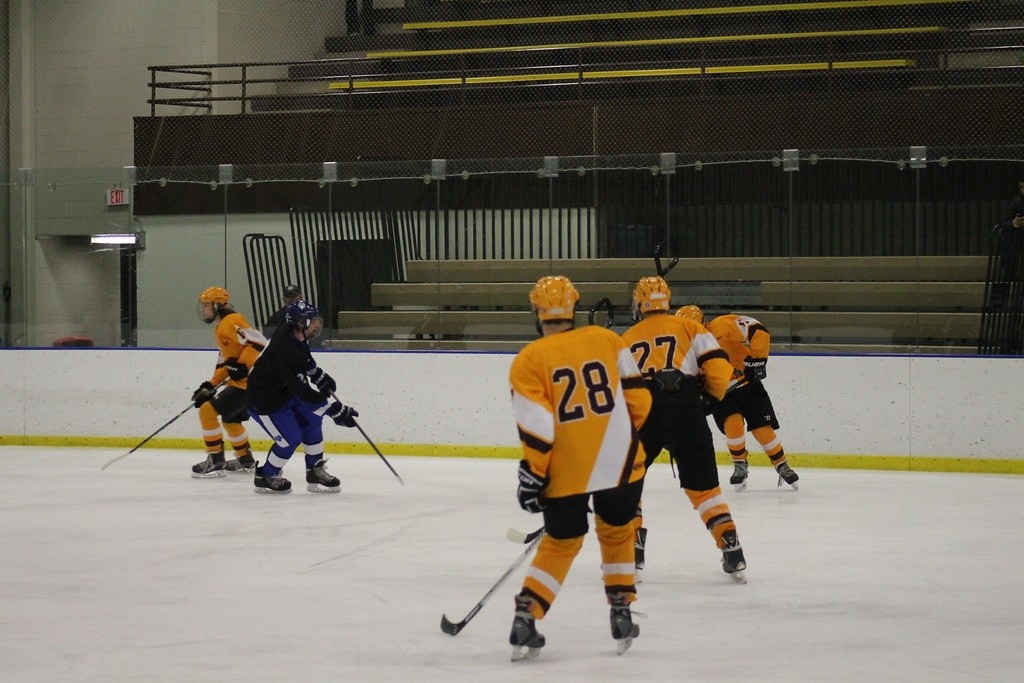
[263,285,303,340]
[619,275,747,573]
[509,275,653,648]
[191,286,267,474]
[674,305,799,485]
[246,299,359,491]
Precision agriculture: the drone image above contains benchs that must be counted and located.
[249,0,1024,355]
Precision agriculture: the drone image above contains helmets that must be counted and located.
[529,276,580,322]
[198,287,229,324]
[286,300,321,329]
[675,305,704,325]
[633,276,671,314]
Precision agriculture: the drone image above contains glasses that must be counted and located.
[285,293,297,299]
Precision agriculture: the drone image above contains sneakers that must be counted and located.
[608,594,638,656]
[509,594,545,662]
[775,460,799,491]
[225,450,256,473]
[253,460,293,494]
[730,458,750,492]
[191,451,227,478]
[306,458,342,493]
[634,527,647,584]
[722,529,748,584]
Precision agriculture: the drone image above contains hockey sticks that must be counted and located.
[441,529,547,636]
[507,524,548,546]
[96,401,197,474]
[353,416,408,485]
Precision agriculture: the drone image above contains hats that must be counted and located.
[283,285,301,296]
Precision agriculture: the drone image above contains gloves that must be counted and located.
[701,393,719,416]
[191,381,217,408]
[517,459,550,513]
[328,402,358,427]
[310,367,336,398]
[744,355,767,383]
[227,362,248,381]
